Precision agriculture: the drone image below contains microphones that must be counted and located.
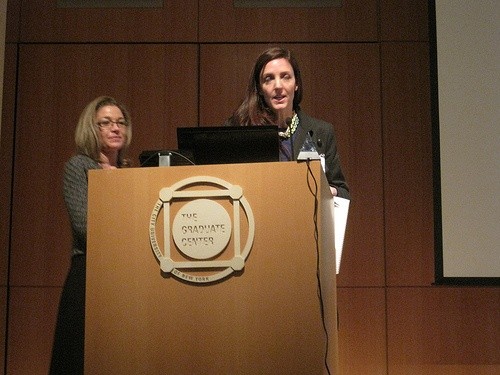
[286,117,294,161]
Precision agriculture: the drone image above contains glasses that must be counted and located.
[97,118,129,127]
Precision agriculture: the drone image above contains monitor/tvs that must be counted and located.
[178,124,279,165]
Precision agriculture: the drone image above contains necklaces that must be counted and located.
[278,113,299,138]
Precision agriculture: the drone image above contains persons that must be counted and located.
[48,95,133,375]
[222,47,351,201]
